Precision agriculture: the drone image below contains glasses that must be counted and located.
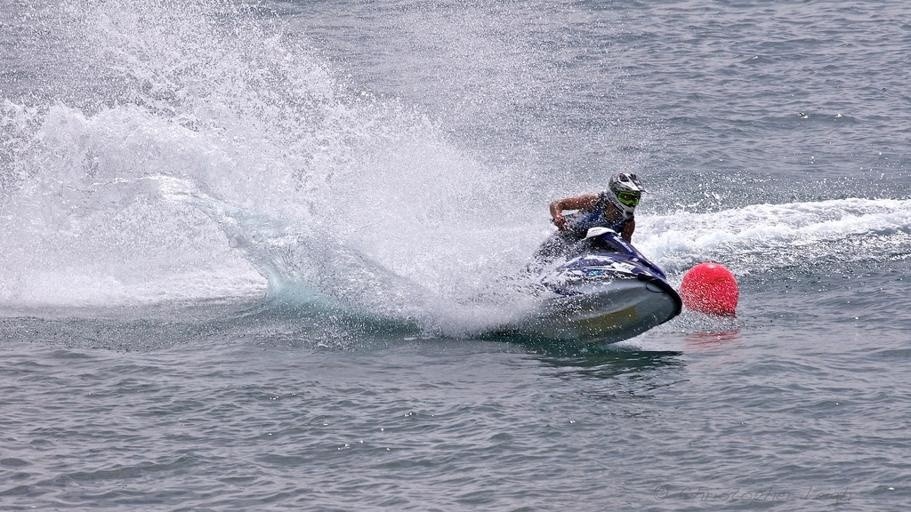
[609,183,641,206]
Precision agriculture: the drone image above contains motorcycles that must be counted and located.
[493,227,684,346]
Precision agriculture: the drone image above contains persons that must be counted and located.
[518,169,642,275]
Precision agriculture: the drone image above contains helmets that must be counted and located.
[605,172,645,220]
